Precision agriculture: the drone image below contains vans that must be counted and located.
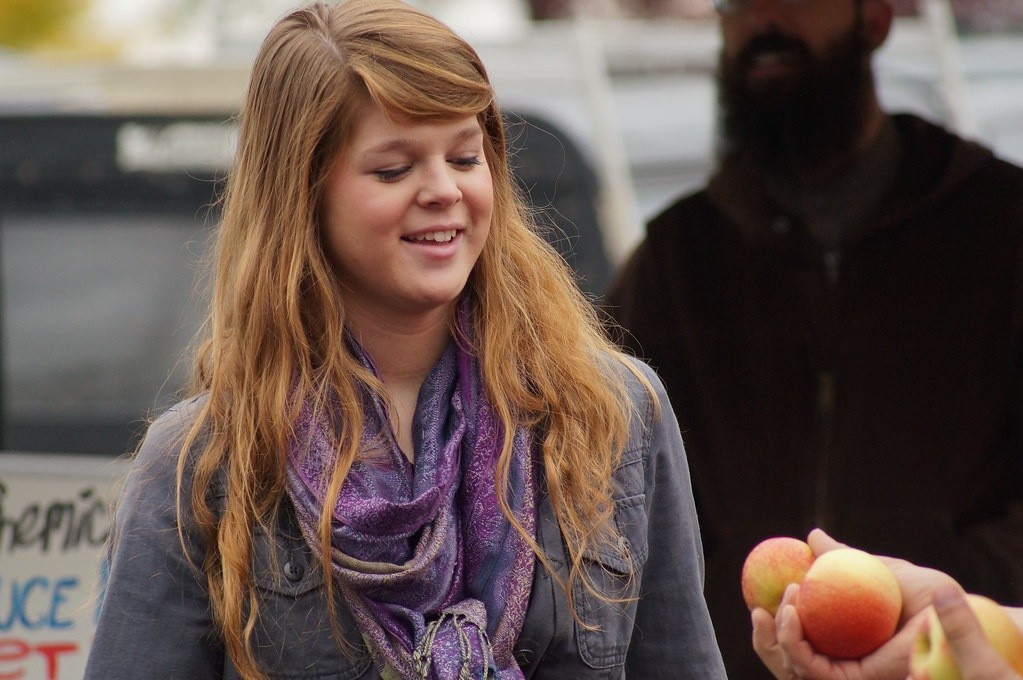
[0,22,646,680]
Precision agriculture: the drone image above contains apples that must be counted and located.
[795,548,901,660]
[742,538,819,620]
[906,593,1023,680]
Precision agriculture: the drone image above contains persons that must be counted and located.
[595,0,1023,680]
[81,0,729,680]
